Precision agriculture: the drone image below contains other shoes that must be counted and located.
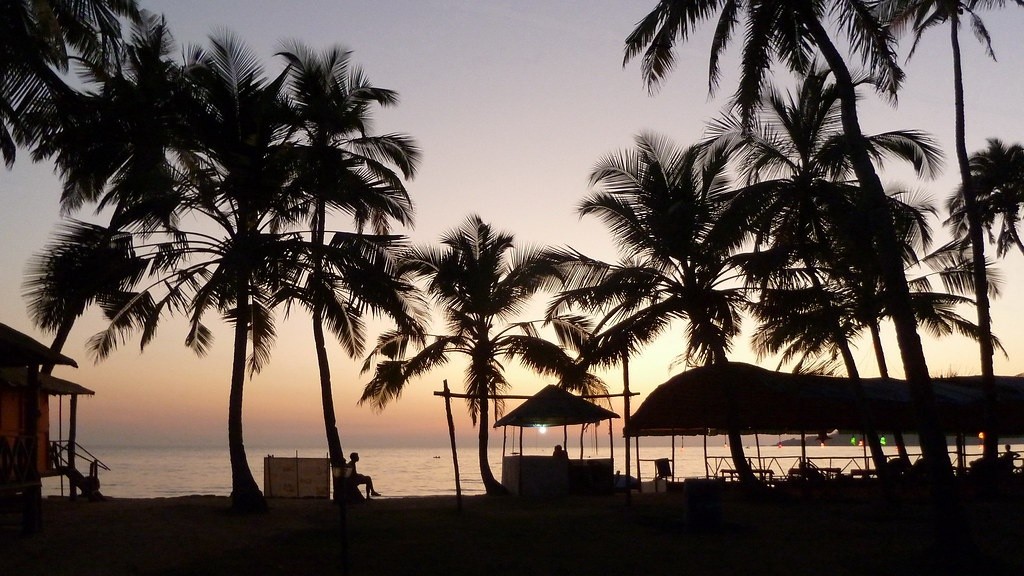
[366,498,373,500]
[371,492,381,496]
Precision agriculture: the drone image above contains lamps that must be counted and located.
[816,433,832,445]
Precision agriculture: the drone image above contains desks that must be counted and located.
[850,469,879,479]
[788,467,841,481]
[720,468,774,486]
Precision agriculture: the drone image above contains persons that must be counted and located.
[552,445,568,458]
[1003,445,1020,471]
[347,453,381,501]
